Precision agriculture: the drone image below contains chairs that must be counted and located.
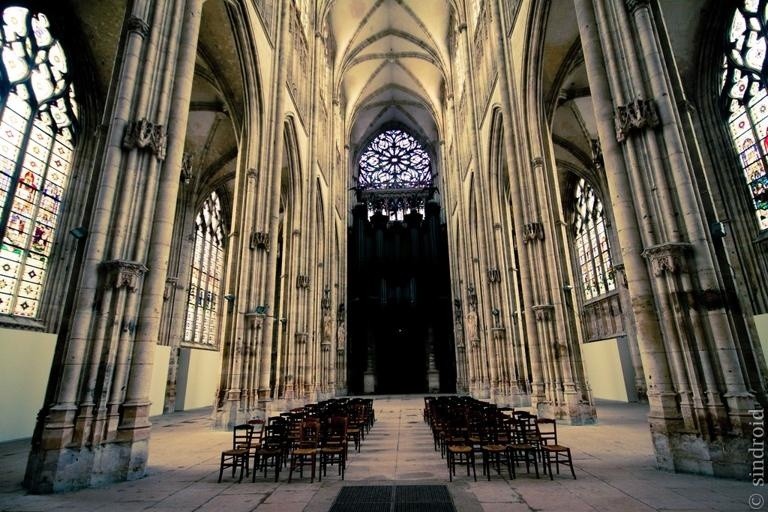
[217,395,376,485]
[421,391,579,483]
